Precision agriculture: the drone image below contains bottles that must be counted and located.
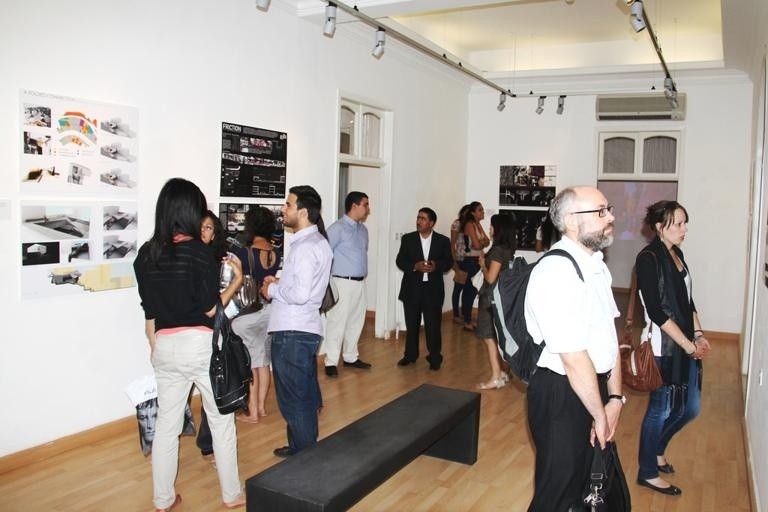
[220,254,232,287]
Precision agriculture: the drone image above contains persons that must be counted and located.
[396,207,454,371]
[477,212,516,390]
[315,215,337,314]
[261,185,333,457]
[451,204,470,324]
[324,191,372,378]
[462,201,491,332]
[134,178,246,512]
[195,209,227,468]
[229,207,281,425]
[536,209,562,253]
[635,199,712,495]
[525,186,626,512]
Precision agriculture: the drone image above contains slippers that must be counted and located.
[236,415,258,423]
[258,412,266,417]
[225,486,245,509]
[154,495,181,512]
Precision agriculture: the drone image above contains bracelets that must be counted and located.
[695,328,704,339]
[687,342,698,359]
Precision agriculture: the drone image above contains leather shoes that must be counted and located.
[201,451,212,457]
[453,318,462,324]
[273,448,292,456]
[637,478,681,496]
[464,324,474,331]
[429,364,439,370]
[325,366,337,378]
[343,360,371,369]
[398,358,416,367]
[656,461,673,474]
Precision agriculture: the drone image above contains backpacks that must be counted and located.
[495,250,585,381]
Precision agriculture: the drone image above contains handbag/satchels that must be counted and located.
[452,271,467,284]
[618,251,662,392]
[208,298,254,416]
[233,246,256,309]
[566,439,632,512]
[470,267,484,291]
[127,374,197,456]
[319,275,338,314]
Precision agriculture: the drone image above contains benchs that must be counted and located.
[246,382,481,511]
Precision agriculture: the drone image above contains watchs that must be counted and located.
[609,393,627,404]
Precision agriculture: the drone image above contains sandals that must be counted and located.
[476,379,504,390]
[500,371,509,382]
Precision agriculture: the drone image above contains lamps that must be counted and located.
[630,0,646,33]
[372,26,385,60]
[323,2,337,37]
[670,89,678,109]
[664,76,673,98]
[535,96,546,114]
[556,95,566,114]
[497,91,506,111]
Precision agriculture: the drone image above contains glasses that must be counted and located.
[570,205,614,218]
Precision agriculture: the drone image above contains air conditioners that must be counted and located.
[596,93,687,121]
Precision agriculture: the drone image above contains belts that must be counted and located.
[548,370,611,384]
[332,275,363,281]
